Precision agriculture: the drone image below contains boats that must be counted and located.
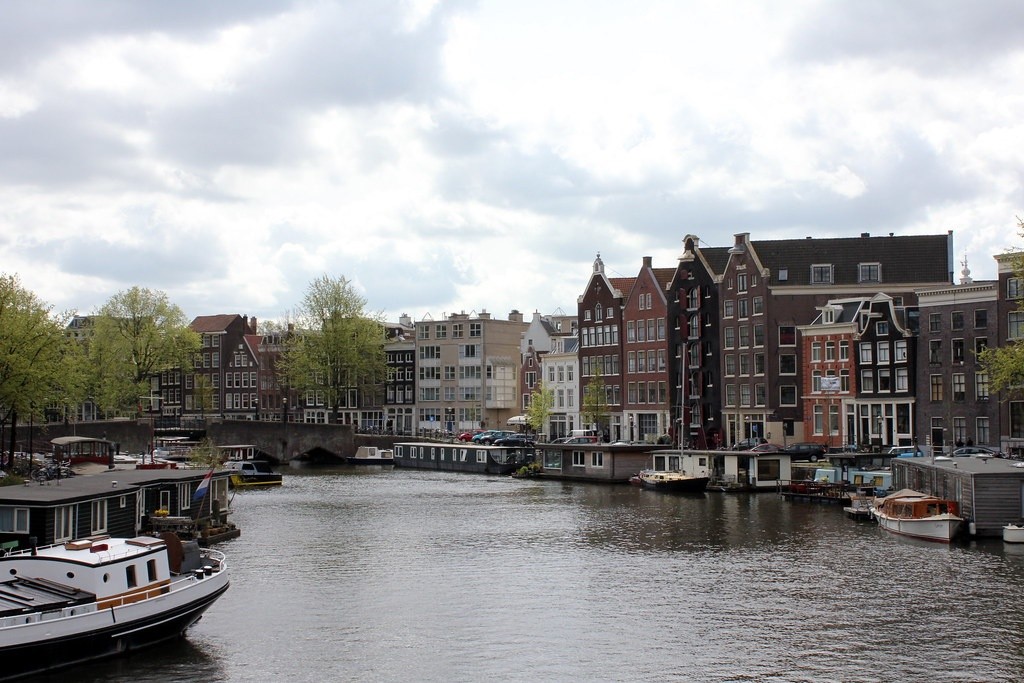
[0,531,229,683]
[869,489,964,543]
[154,436,283,488]
[346,446,394,464]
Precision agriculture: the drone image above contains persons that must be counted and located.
[956,436,973,453]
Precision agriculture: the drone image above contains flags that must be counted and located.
[194,468,212,496]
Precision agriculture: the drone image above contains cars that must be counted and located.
[887,446,923,458]
[472,430,497,444]
[731,438,768,451]
[551,430,597,444]
[480,431,516,446]
[458,429,485,443]
[751,444,784,452]
[947,447,1009,458]
[494,434,534,446]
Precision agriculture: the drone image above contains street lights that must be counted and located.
[254,398,259,420]
[448,406,452,431]
[283,398,287,422]
[876,415,883,453]
[524,413,528,446]
[744,416,750,450]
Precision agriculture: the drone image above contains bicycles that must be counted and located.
[30,461,71,481]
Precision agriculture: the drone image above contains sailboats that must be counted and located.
[637,342,711,492]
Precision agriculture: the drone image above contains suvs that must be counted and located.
[780,442,827,463]
